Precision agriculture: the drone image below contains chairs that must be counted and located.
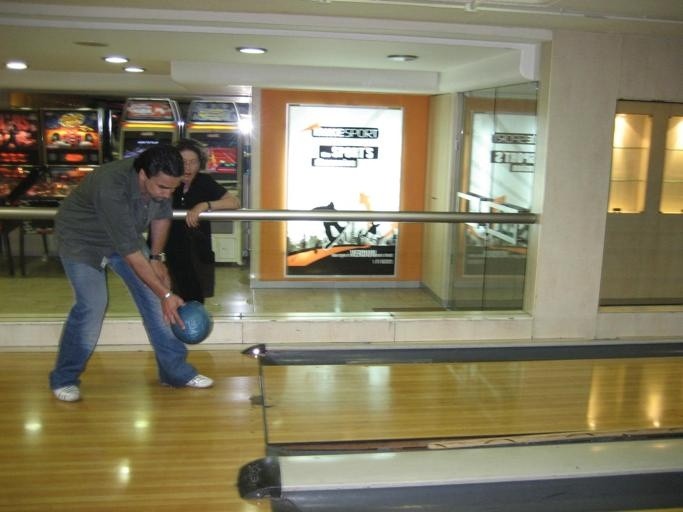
[0,197,22,276]
[19,200,62,276]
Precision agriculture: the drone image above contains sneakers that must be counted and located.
[160,373,213,389]
[52,383,81,402]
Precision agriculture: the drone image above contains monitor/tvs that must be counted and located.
[124,141,161,159]
[197,140,237,175]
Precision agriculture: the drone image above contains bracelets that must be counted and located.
[148,252,167,263]
[205,201,212,211]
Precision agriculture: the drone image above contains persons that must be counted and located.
[141,136,244,309]
[47,142,216,403]
